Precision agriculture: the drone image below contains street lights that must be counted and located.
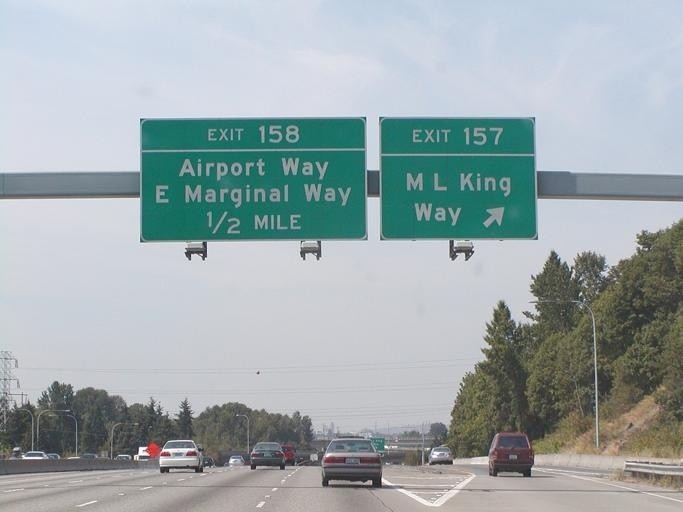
[398,424,425,465]
[48,414,78,456]
[36,408,71,451]
[109,422,140,460]
[1,406,34,450]
[235,414,249,453]
[528,298,601,446]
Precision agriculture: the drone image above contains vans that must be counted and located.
[485,430,532,477]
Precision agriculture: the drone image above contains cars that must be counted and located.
[428,445,452,465]
[157,438,298,473]
[10,446,150,461]
[320,436,382,488]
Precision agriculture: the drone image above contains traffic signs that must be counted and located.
[376,114,537,245]
[137,118,366,241]
[367,435,384,451]
